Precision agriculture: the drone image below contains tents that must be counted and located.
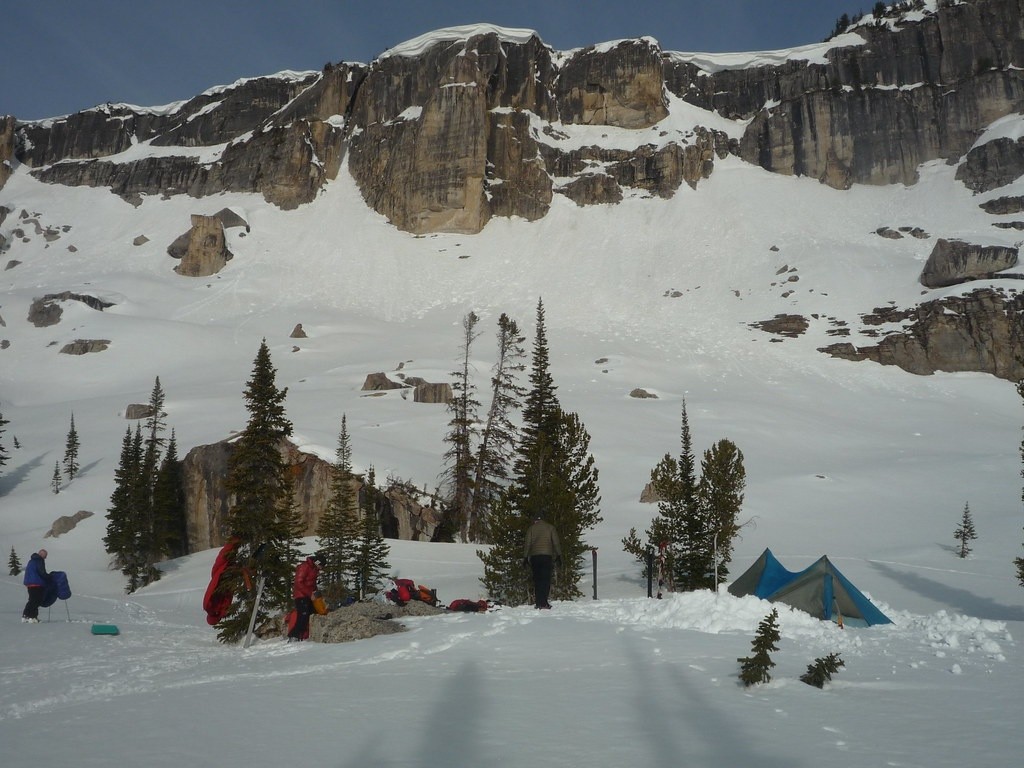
[766,555,898,627]
[728,547,794,600]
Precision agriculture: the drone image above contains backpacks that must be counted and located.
[450,599,488,611]
[387,578,441,607]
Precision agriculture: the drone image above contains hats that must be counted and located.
[314,554,328,566]
[535,512,546,520]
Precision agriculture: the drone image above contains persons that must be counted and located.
[523,510,564,610]
[21,549,49,623]
[287,555,325,640]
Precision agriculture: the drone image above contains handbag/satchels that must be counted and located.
[312,593,329,615]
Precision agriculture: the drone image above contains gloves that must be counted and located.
[523,557,529,567]
[554,556,562,566]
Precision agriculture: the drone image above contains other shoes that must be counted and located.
[539,604,552,609]
[22,616,43,624]
